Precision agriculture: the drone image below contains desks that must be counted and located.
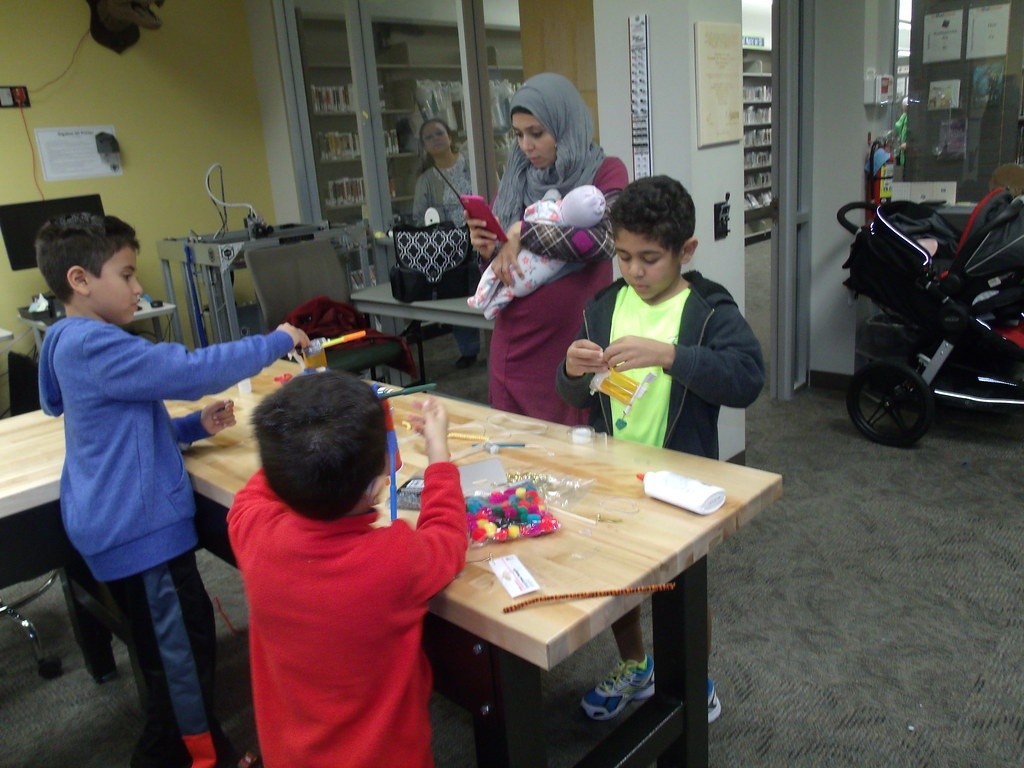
[17,297,183,357]
[0,359,784,768]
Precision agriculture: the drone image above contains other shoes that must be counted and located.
[237,751,262,768]
[456,356,477,370]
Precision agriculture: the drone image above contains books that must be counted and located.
[742,85,772,103]
[743,106,771,124]
[744,191,772,211]
[310,83,385,113]
[744,151,771,168]
[327,176,396,206]
[744,172,771,187]
[744,129,772,148]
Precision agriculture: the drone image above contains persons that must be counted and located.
[894,97,908,182]
[35,212,313,768]
[553,175,767,725]
[467,185,606,320]
[226,371,468,768]
[412,119,501,368]
[316,129,399,160]
[463,72,628,427]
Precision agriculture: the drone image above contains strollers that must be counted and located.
[836,187,1024,446]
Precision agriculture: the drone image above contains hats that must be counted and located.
[561,184,606,229]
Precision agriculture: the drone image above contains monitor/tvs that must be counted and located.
[0,193,105,271]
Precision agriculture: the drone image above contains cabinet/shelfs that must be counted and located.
[743,45,772,239]
[348,280,498,381]
[296,13,525,229]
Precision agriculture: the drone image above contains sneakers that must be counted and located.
[706,676,721,724]
[579,654,655,722]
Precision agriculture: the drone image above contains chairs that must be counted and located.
[244,236,404,382]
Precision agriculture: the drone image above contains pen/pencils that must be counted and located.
[376,383,437,401]
[322,329,366,349]
[469,441,525,448]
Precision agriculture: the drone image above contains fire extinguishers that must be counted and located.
[864,129,896,219]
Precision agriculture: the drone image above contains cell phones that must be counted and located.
[460,194,508,242]
